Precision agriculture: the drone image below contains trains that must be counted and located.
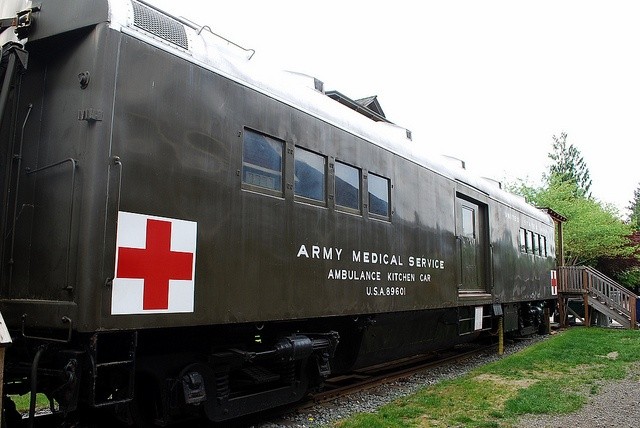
[0,0,559,425]
